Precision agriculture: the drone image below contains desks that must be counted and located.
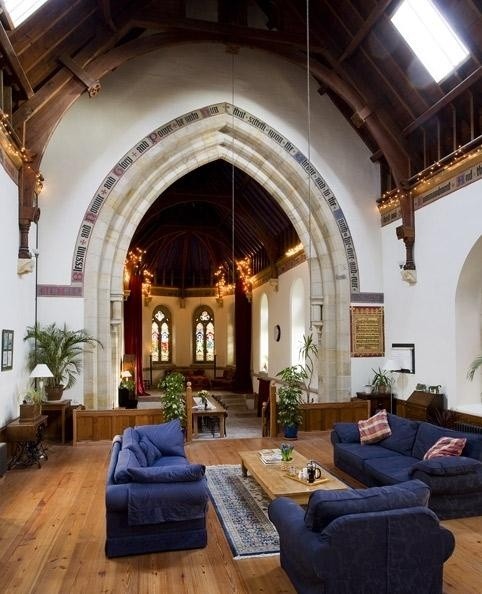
[6,416,48,469]
[192,395,226,438]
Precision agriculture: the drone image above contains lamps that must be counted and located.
[29,364,53,402]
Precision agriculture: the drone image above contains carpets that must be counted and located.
[205,465,357,560]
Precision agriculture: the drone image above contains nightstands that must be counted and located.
[377,413,422,456]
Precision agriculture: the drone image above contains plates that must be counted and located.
[284,472,330,485]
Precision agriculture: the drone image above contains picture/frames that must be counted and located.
[3,329,13,370]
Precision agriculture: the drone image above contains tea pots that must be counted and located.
[306,460,321,479]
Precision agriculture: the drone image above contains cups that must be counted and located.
[303,468,308,477]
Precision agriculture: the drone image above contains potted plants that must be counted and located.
[24,321,103,401]
[281,445,295,470]
[275,366,308,437]
[366,367,394,393]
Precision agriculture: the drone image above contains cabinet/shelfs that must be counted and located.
[358,392,395,417]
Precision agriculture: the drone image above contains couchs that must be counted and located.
[330,410,482,521]
[104,419,209,559]
[269,479,455,591]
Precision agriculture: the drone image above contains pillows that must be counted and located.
[140,436,160,465]
[408,456,482,476]
[411,421,482,460]
[332,424,359,443]
[424,437,466,462]
[126,463,206,483]
[357,410,393,447]
[133,420,187,458]
